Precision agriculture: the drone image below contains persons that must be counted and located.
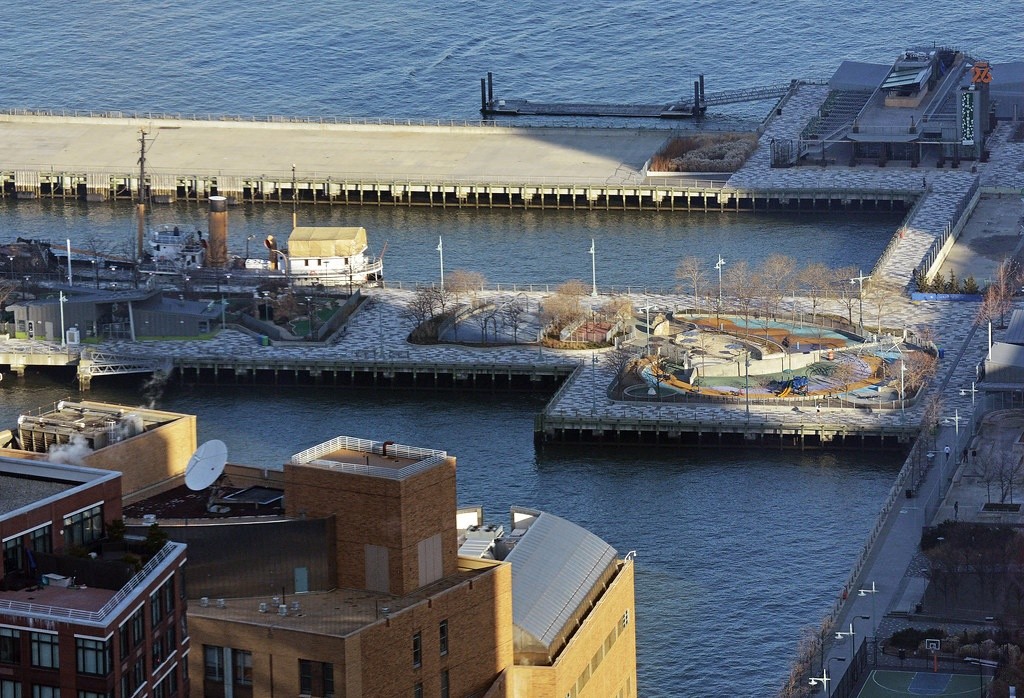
[944,444,952,461]
[962,447,969,463]
[972,447,977,465]
[4,320,8,335]
[815,401,821,418]
[782,336,790,348]
[953,501,959,519]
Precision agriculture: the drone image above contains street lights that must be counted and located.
[827,657,847,696]
[900,360,909,415]
[849,269,873,328]
[436,235,446,290]
[833,623,856,640]
[858,580,878,637]
[958,381,977,438]
[941,409,961,465]
[588,238,599,297]
[714,254,727,308]
[808,668,830,692]
[927,449,944,499]
[985,615,1006,652]
[963,658,983,697]
[743,353,754,419]
[898,505,925,532]
[853,615,871,659]
[590,299,656,414]
[57,291,68,347]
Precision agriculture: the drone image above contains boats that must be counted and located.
[105,126,386,287]
